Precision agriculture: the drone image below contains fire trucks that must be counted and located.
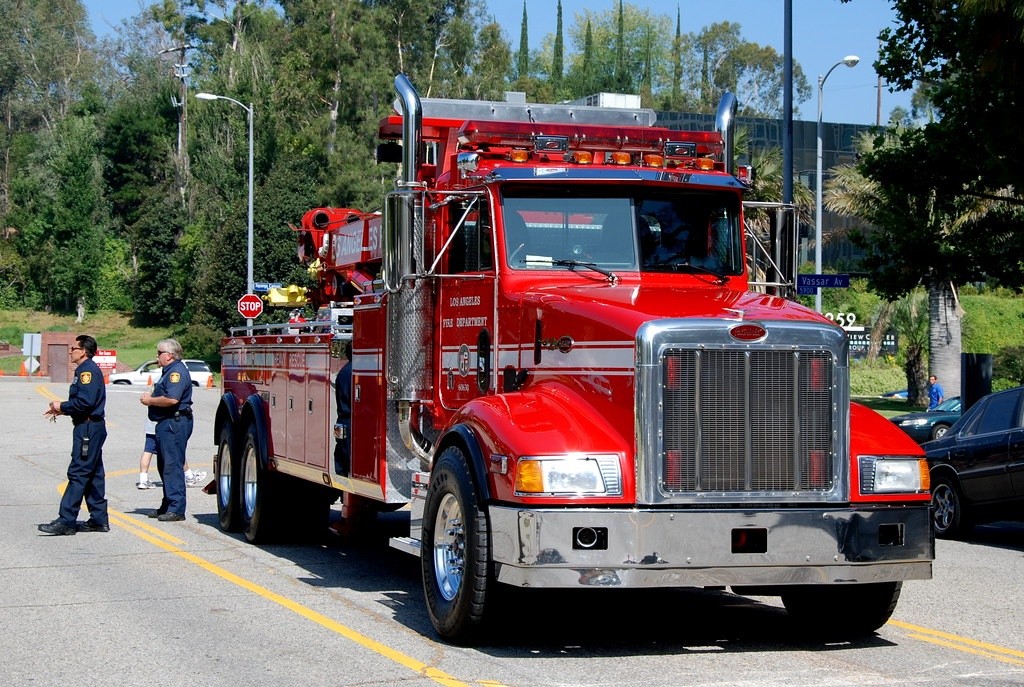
[203,73,934,645]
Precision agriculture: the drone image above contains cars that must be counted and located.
[108,358,213,389]
[918,385,1023,537]
[888,395,961,441]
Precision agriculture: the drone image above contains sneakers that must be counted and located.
[38,520,76,536]
[77,521,109,532]
[148,508,168,518]
[184,471,207,487]
[138,482,155,490]
[159,510,185,521]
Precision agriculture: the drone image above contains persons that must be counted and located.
[334,340,353,481]
[141,338,193,521]
[139,368,207,489]
[926,375,943,412]
[38,335,110,535]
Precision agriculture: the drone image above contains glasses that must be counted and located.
[71,346,84,351]
[157,351,173,356]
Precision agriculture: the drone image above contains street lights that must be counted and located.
[815,55,860,311]
[196,91,255,333]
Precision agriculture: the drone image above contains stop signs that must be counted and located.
[238,294,263,319]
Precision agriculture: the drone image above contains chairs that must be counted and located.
[473,206,527,271]
